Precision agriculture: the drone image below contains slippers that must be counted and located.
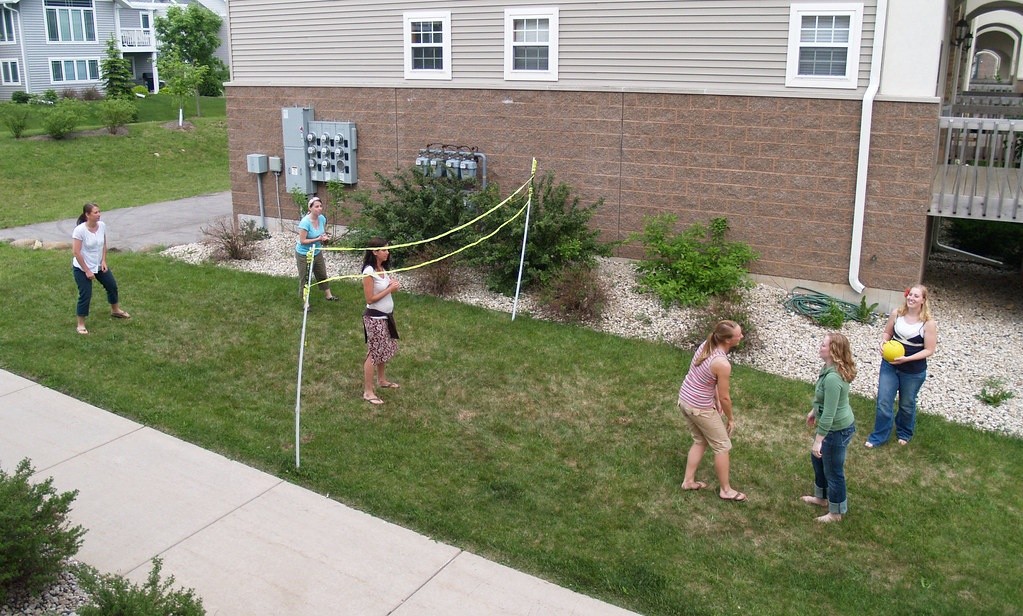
[379,382,400,388]
[303,306,311,312]
[111,312,130,318]
[327,296,338,300]
[686,481,708,490]
[364,397,385,405]
[77,327,89,336]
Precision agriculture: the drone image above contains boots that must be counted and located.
[722,492,747,502]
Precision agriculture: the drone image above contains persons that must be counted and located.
[361,239,401,404]
[864,284,937,448]
[800,334,858,522]
[677,320,747,502]
[72,203,130,335]
[295,196,339,311]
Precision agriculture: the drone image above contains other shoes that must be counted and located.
[865,442,873,449]
[802,495,828,507]
[815,514,840,525]
[899,439,907,445]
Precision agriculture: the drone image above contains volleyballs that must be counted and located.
[882,339,905,363]
